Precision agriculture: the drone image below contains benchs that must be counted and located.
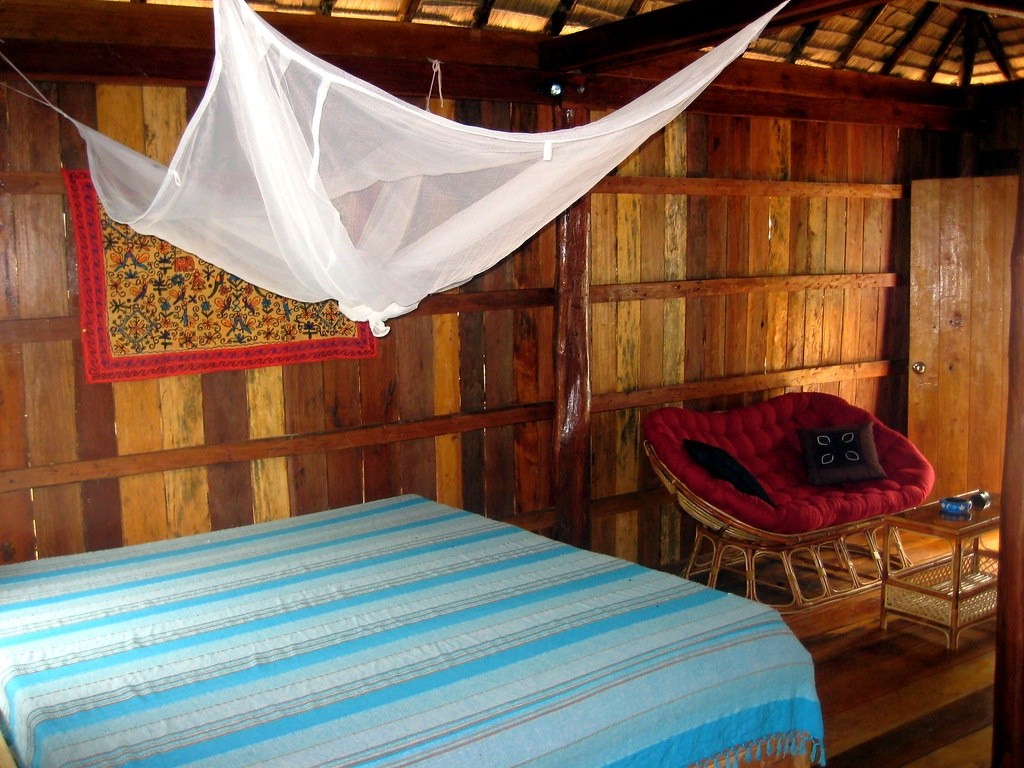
[641,392,936,614]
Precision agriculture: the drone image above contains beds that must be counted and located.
[0,493,825,767]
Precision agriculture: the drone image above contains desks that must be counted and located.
[880,489,1001,652]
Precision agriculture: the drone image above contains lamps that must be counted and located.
[541,78,562,97]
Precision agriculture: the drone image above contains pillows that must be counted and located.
[794,420,888,485]
[682,438,774,508]
